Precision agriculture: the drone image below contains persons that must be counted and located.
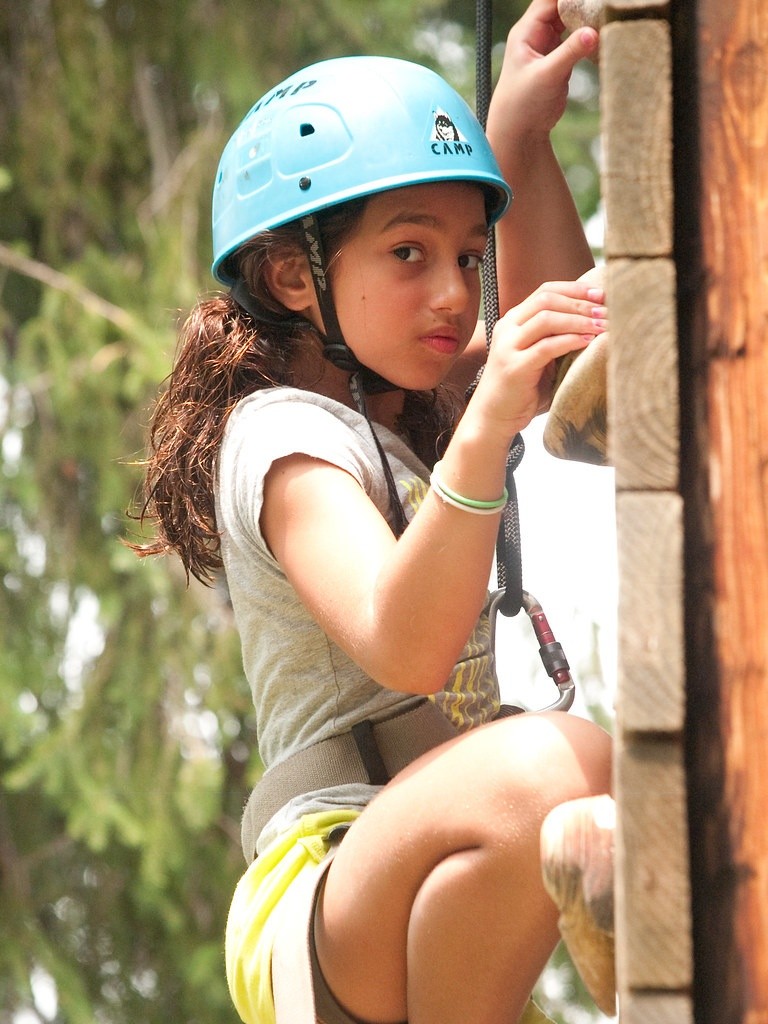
[143,0,609,1024]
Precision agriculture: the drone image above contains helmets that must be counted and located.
[211,56,514,287]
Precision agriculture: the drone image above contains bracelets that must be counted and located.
[430,462,507,514]
